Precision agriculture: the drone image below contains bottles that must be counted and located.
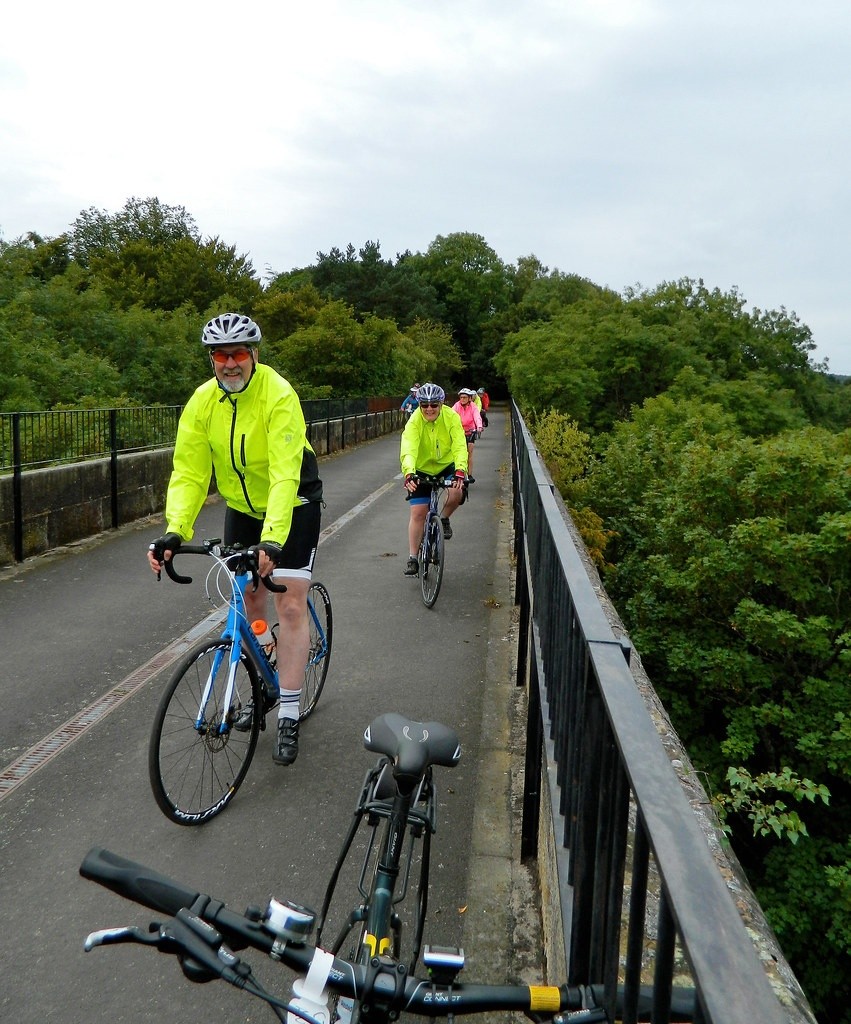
[251,621,278,663]
[334,996,354,1024]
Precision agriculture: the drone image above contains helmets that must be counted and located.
[410,386,418,391]
[477,389,484,393]
[201,312,262,344]
[417,383,445,403]
[458,388,476,395]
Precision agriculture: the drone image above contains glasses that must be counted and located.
[211,350,251,363]
[420,403,439,408]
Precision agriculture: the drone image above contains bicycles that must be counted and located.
[77,713,705,1024]
[405,473,476,609]
[147,538,334,826]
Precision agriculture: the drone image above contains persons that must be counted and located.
[147,313,326,758]
[398,383,490,577]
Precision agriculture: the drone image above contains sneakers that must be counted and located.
[234,696,266,732]
[440,517,453,540]
[272,717,299,767]
[468,475,475,483]
[403,557,419,577]
